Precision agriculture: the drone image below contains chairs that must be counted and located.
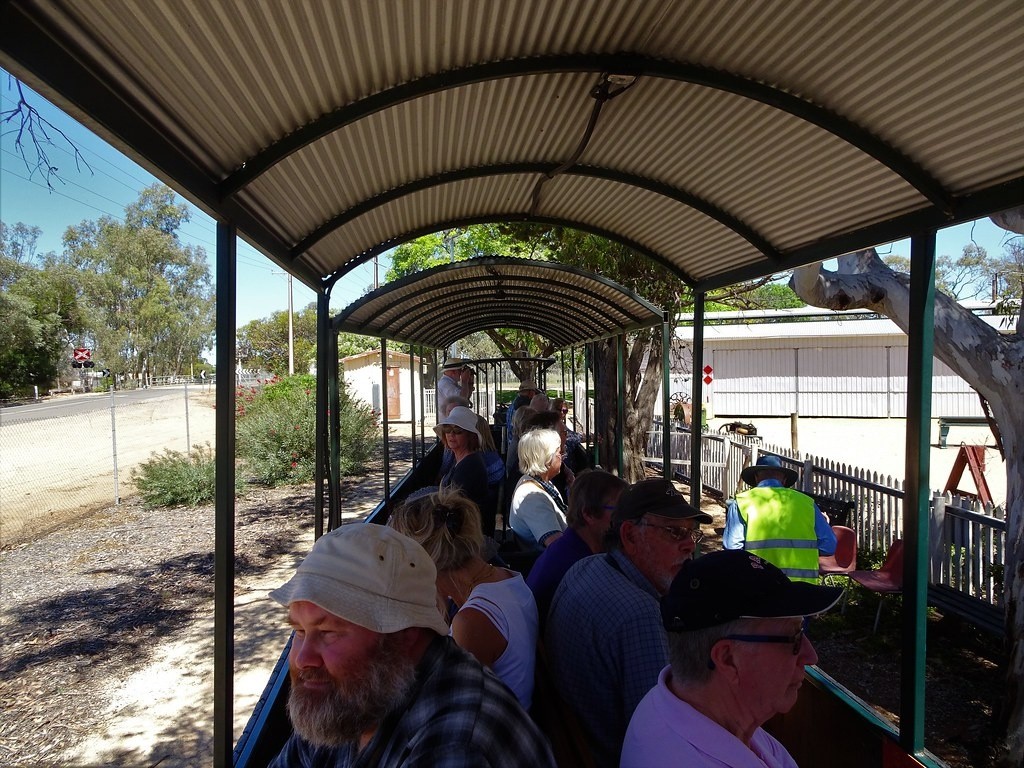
[841,538,904,635]
[819,524,862,612]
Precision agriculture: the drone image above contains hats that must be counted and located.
[269,522,451,636]
[741,456,798,487]
[613,477,713,524]
[517,380,536,393]
[433,406,482,447]
[441,358,465,372]
[660,549,844,632]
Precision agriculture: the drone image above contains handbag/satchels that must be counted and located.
[498,403,507,423]
[479,449,505,485]
[574,443,593,472]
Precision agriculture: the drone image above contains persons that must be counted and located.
[265,520,558,768]
[617,548,849,768]
[384,357,715,711]
[720,452,840,585]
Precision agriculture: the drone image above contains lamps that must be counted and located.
[605,55,640,85]
[493,289,506,299]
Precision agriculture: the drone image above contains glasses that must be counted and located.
[647,523,705,544]
[557,408,568,414]
[707,627,805,670]
[443,425,466,434]
